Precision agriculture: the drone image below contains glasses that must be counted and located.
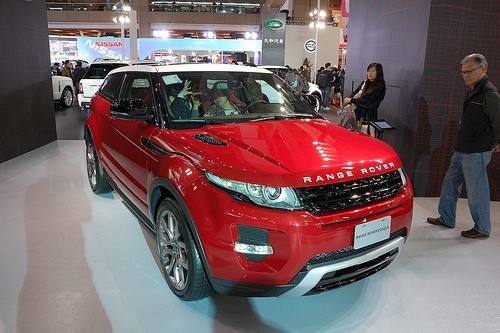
[462,65,482,74]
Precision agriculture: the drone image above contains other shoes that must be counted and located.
[322,106,330,110]
[321,106,323,108]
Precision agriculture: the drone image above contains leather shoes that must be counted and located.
[427,217,455,228]
[461,228,489,238]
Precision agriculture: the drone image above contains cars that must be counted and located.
[82,66,413,303]
[132,61,164,66]
[94,58,120,62]
[70,59,89,69]
[51,71,74,108]
[260,66,323,114]
[78,62,129,110]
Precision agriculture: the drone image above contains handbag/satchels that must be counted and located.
[352,80,367,99]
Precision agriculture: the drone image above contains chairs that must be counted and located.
[168,80,247,116]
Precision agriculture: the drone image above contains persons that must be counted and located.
[427,53,500,238]
[171,0,223,13]
[52,57,386,125]
